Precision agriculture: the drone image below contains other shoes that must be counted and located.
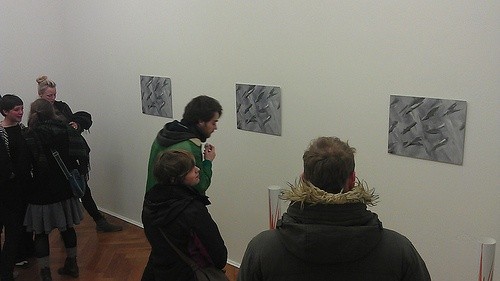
[96,216,122,232]
[15,257,32,269]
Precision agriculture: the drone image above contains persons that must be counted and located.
[13,98,90,281]
[141,150,228,281]
[36,74,124,233]
[237,136,432,281]
[141,95,222,281]
[0,94,29,281]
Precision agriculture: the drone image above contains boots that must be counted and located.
[39,266,52,281]
[57,256,79,278]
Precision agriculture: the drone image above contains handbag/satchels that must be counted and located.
[67,169,86,200]
[193,267,230,281]
